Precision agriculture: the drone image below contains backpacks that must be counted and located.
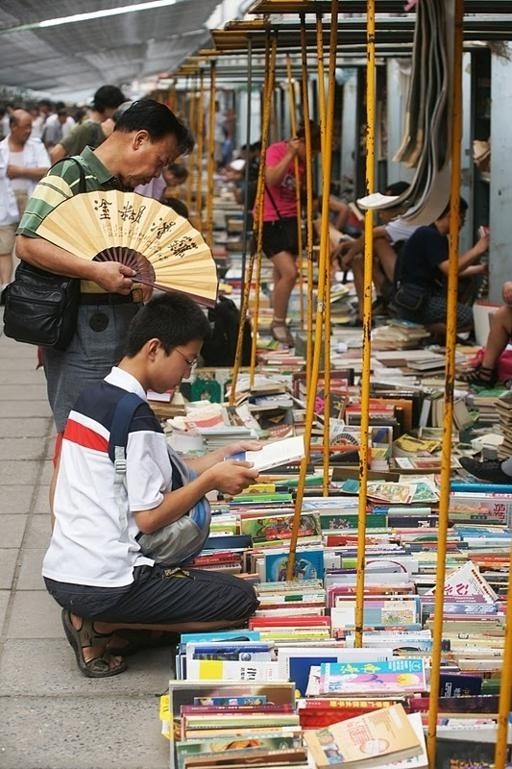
[135,443,214,572]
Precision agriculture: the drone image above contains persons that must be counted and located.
[0,78,490,329]
[14,98,198,533]
[252,118,323,349]
[37,290,265,680]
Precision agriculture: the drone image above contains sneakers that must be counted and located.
[338,317,375,328]
[458,457,512,485]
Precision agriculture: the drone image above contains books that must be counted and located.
[150,176,510,768]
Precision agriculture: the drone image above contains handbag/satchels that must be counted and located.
[276,221,299,255]
[0,259,81,349]
[393,284,425,312]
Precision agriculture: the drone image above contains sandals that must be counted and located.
[269,320,295,347]
[455,362,498,388]
[60,606,153,679]
